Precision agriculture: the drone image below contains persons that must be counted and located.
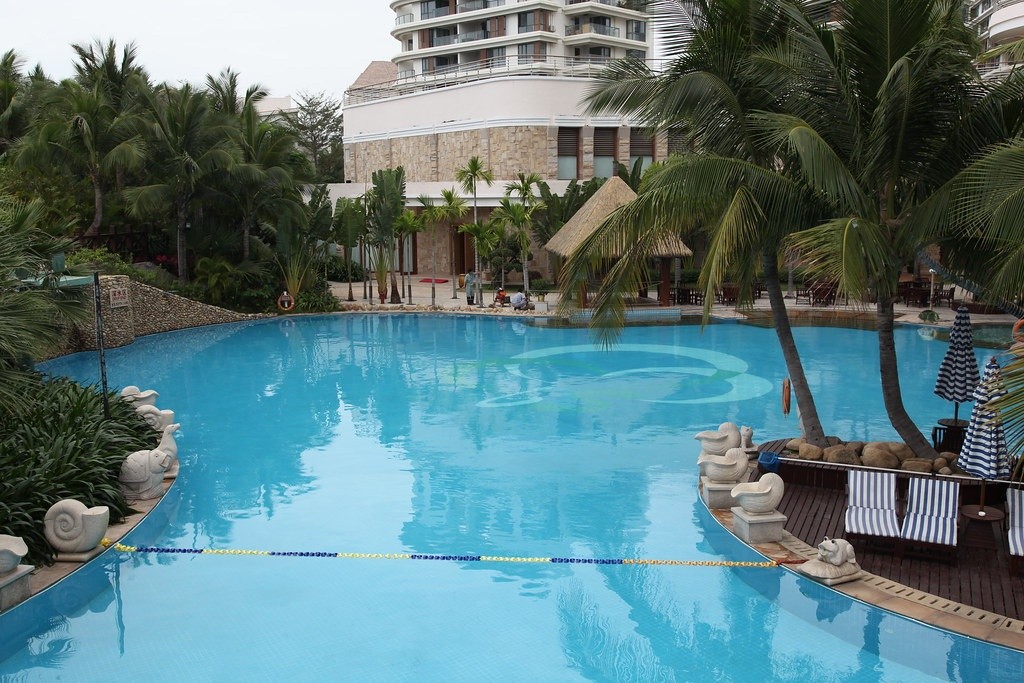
[463,268,476,306]
[511,288,527,311]
[494,287,507,307]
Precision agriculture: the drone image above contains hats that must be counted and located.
[518,289,523,292]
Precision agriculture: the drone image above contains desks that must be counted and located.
[958,505,1007,562]
[938,419,969,428]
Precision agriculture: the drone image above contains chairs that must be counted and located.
[900,477,960,561]
[932,427,968,453]
[845,470,900,556]
[671,277,955,309]
[1006,488,1024,578]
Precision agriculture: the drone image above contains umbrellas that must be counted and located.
[933,302,981,420]
[956,356,1010,511]
